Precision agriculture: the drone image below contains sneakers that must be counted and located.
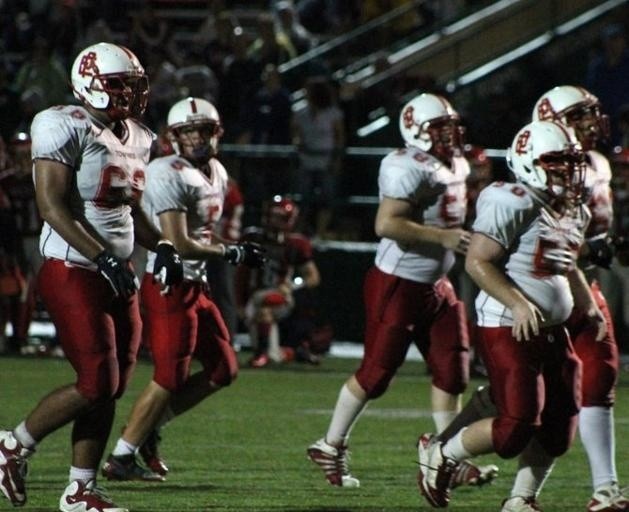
[103,453,164,483]
[500,496,542,511]
[0,430,35,507]
[122,427,168,474]
[417,433,455,508]
[60,478,128,512]
[586,483,628,512]
[449,457,497,489]
[308,438,360,488]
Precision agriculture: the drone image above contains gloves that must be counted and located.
[220,241,264,267]
[587,238,615,270]
[93,246,135,297]
[151,241,182,296]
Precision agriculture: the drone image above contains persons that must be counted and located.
[100,97,267,481]
[0,0,629,366]
[0,41,183,512]
[437,84,629,512]
[306,93,500,489]
[415,119,606,512]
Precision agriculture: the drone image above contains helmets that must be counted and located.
[510,122,585,202]
[400,93,464,159]
[168,97,221,160]
[532,86,606,153]
[71,42,149,119]
[264,196,298,231]
